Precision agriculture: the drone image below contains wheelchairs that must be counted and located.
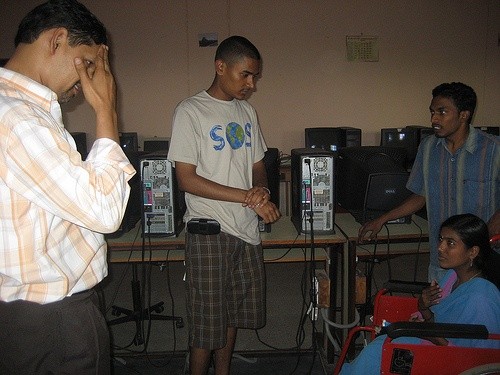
[339,234,500,372]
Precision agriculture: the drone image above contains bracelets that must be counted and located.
[262,187,271,193]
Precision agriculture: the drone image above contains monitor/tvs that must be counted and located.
[257,147,280,234]
[335,144,414,227]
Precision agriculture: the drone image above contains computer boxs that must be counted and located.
[288,148,335,236]
[139,148,184,237]
[302,126,361,189]
[378,126,435,172]
[102,131,143,239]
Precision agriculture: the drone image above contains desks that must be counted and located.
[106,213,429,364]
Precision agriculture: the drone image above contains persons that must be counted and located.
[167,35,281,375]
[0,0,137,375]
[357,82,500,283]
[338,213,500,375]
